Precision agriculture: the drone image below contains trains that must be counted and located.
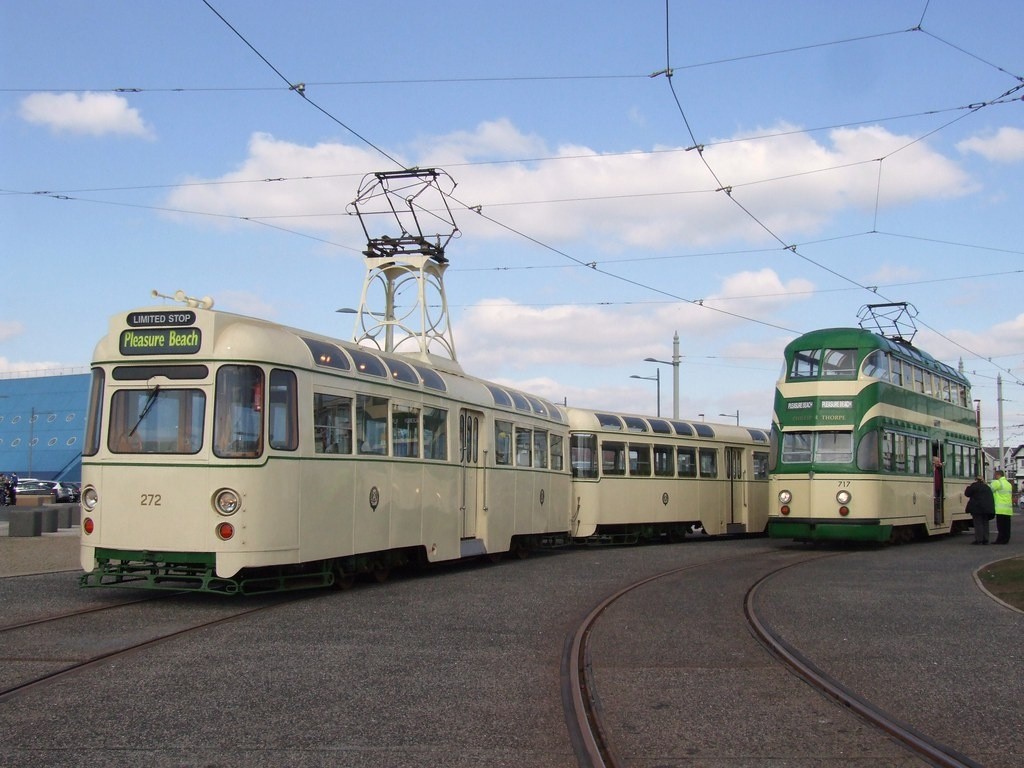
[64,155,986,608]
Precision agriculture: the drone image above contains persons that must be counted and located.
[1,470,18,505]
[988,471,1013,543]
[964,475,996,544]
[932,454,944,523]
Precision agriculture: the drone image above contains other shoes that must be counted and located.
[972,539,989,544]
[990,541,1003,544]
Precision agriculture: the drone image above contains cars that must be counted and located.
[1,467,82,507]
